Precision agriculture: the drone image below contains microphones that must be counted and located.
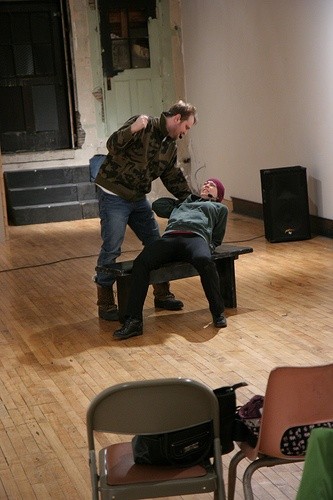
[207,194,218,201]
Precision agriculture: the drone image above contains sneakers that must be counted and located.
[113,320,142,338]
[212,312,227,327]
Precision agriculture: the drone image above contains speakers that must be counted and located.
[260,166,312,243]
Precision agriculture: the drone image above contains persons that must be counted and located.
[94,99,197,320]
[113,179,229,339]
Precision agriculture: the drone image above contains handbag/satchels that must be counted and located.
[233,394,333,456]
[131,382,248,469]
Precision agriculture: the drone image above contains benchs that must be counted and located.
[95,241,254,325]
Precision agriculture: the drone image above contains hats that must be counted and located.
[207,177,224,202]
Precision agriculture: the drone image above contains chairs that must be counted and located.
[86,378,227,500]
[227,362,333,500]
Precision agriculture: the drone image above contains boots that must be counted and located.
[97,285,120,321]
[153,282,182,310]
